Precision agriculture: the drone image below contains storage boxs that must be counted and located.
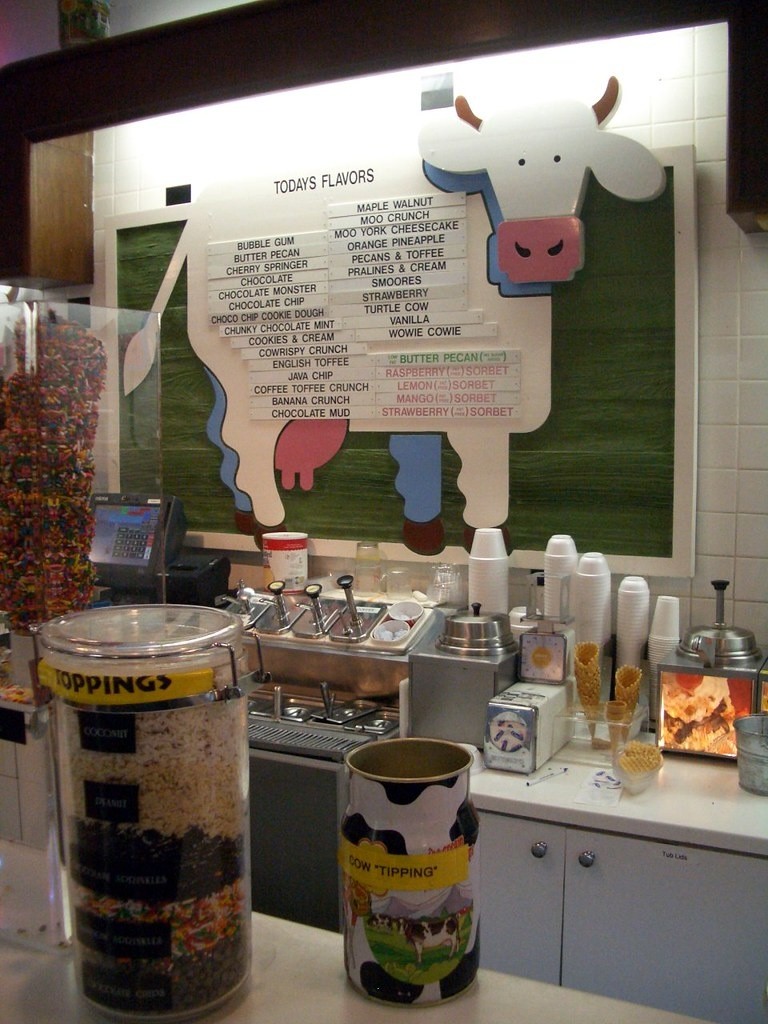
[484,680,573,775]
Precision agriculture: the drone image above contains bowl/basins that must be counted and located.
[508,606,542,628]
[389,600,424,627]
[467,527,510,615]
[373,620,411,642]
[543,535,650,721]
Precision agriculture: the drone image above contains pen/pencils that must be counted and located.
[527,767,569,787]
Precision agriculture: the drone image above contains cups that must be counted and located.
[647,596,680,720]
[388,563,411,601]
[40,602,254,1024]
[353,541,387,597]
[339,737,482,1009]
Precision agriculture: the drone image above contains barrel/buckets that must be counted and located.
[732,713,768,796]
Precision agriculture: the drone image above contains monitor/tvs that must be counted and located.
[88,492,188,591]
